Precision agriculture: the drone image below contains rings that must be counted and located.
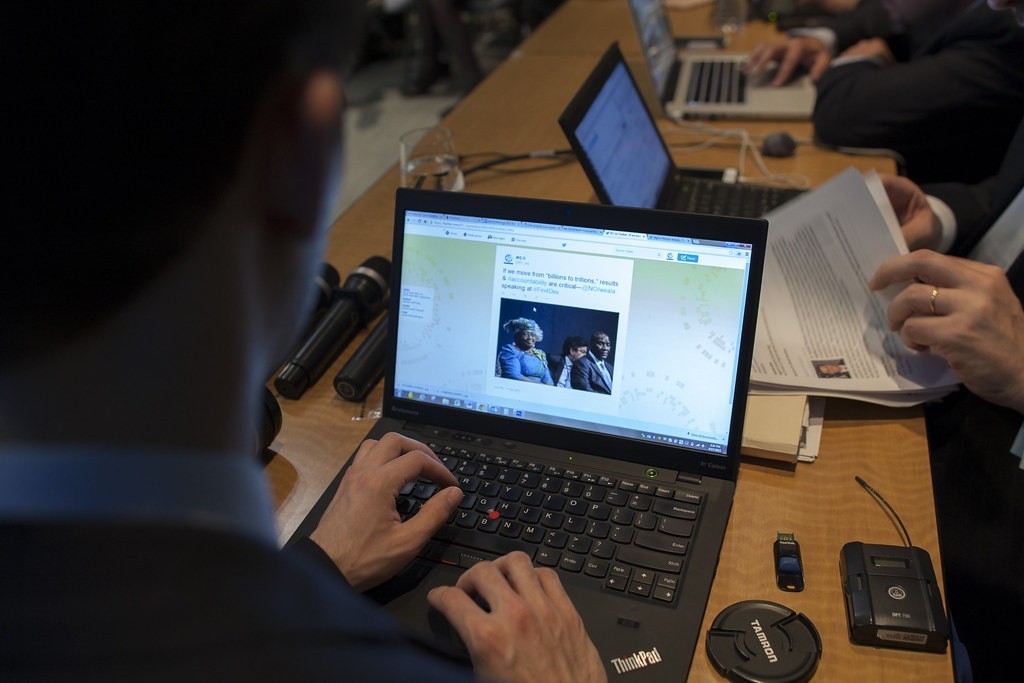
[929,286,938,314]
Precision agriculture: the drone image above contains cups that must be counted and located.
[707,1,748,49]
[399,126,465,193]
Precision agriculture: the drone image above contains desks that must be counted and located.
[256,0,957,682]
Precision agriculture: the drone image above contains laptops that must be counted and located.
[628,0,818,122]
[557,40,812,219]
[280,185,770,681]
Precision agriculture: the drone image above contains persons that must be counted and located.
[495,317,555,386]
[552,336,588,389]
[1,0,608,682]
[741,1,1023,151]
[866,153,1024,478]
[817,359,851,379]
[570,330,613,395]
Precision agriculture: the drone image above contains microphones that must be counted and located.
[281,263,341,366]
[271,255,392,401]
[333,309,392,403]
